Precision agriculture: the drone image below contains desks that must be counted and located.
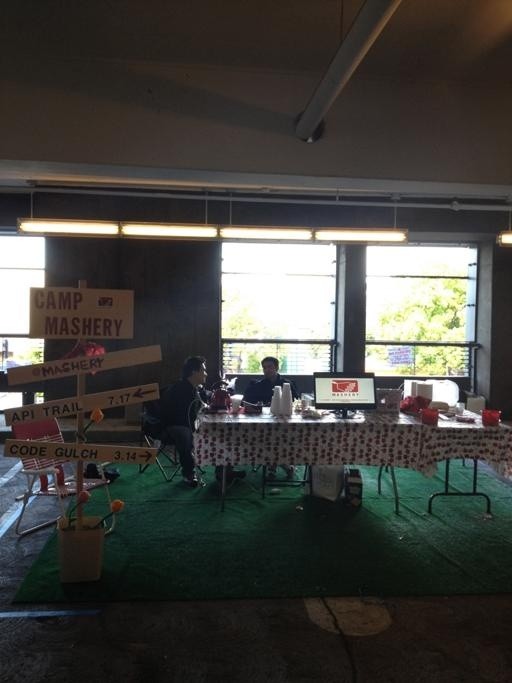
[195,395,512,521]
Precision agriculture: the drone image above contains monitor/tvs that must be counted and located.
[313,372,377,419]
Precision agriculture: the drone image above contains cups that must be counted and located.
[421,408,438,425]
[482,409,501,427]
[456,403,465,416]
[231,396,242,411]
[270,382,293,416]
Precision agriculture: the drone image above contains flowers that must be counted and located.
[57,486,124,530]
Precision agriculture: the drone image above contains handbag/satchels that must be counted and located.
[305,462,344,502]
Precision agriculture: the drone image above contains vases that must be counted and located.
[58,517,104,584]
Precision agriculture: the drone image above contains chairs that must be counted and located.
[11,416,117,536]
[138,386,185,481]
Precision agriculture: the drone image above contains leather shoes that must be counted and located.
[184,472,198,487]
[216,468,246,479]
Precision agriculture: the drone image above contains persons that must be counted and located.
[142,356,214,488]
[241,355,297,478]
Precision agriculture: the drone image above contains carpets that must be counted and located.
[9,453,511,607]
